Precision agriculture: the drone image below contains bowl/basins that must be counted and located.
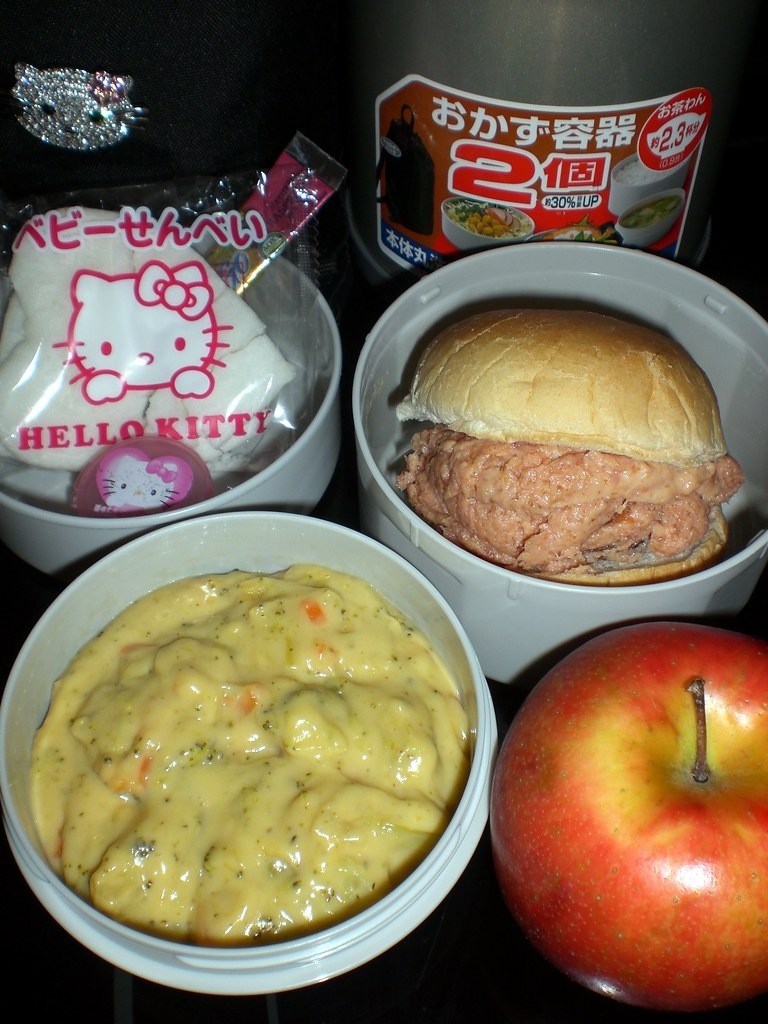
[352,242,768,686]
[0,184,342,578]
[0,511,498,996]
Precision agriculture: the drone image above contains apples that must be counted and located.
[488,621,768,1012]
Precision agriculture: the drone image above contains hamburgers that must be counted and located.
[396,306,742,584]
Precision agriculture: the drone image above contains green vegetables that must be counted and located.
[445,199,495,222]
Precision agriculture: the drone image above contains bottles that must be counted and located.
[343,0,737,303]
